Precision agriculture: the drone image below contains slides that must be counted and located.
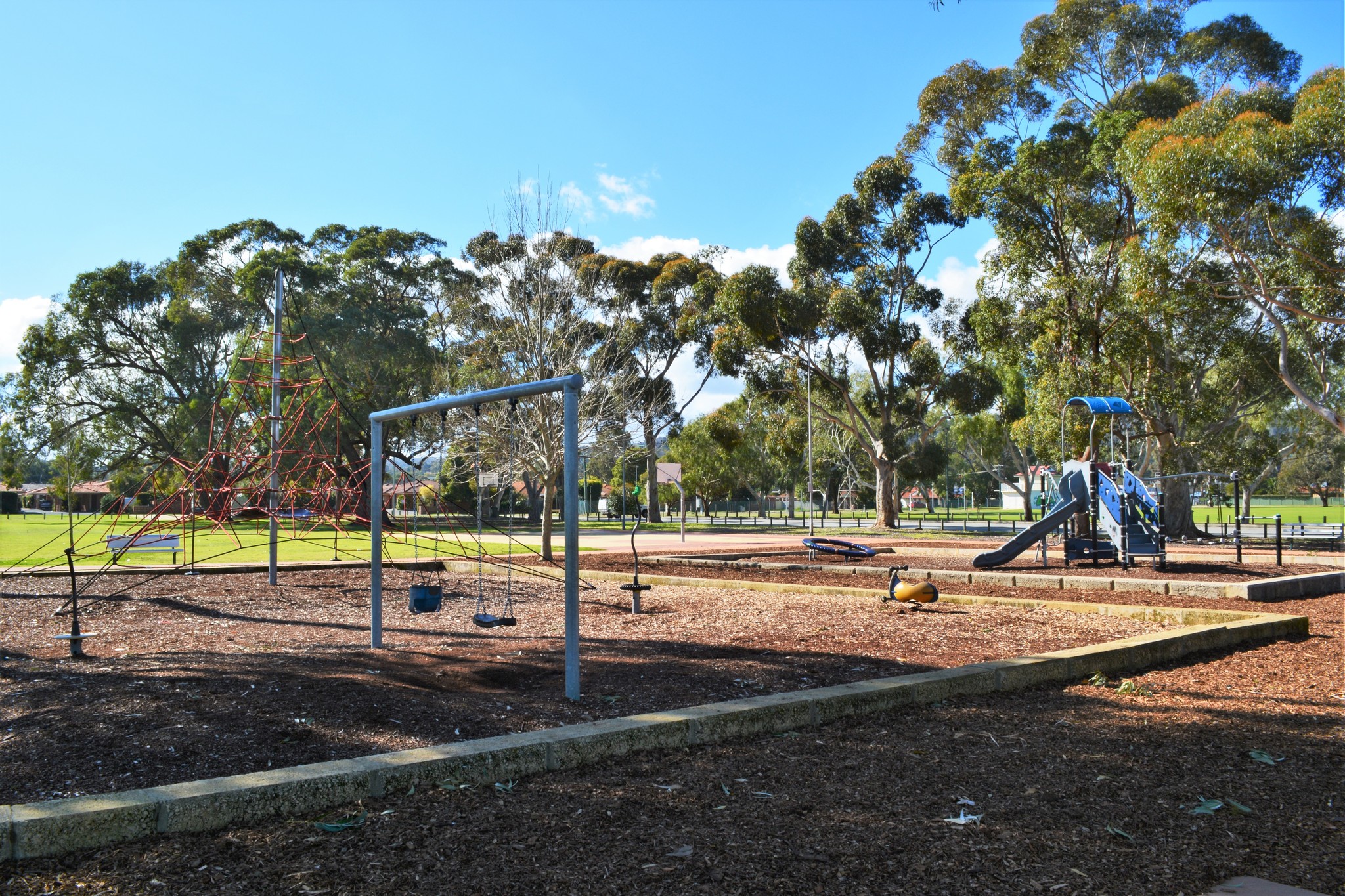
[972,473,1087,572]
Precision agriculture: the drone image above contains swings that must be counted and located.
[471,397,519,631]
[409,410,443,619]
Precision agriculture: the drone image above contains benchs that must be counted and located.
[1281,523,1343,552]
[106,535,184,564]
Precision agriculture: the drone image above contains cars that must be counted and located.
[1146,486,1163,501]
[39,500,52,510]
[1189,491,1219,502]
[1117,484,1125,493]
[907,502,926,509]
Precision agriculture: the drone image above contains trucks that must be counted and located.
[120,497,137,510]
[598,495,648,518]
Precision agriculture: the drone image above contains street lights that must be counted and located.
[636,466,639,489]
[943,427,950,521]
[994,464,1004,508]
[583,453,588,514]
